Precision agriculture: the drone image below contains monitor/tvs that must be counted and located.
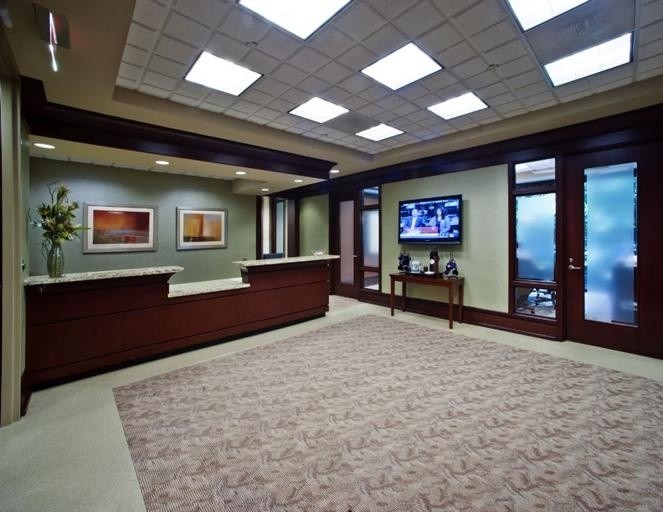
[398,194,462,245]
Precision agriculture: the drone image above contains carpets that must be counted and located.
[110,312,661,512]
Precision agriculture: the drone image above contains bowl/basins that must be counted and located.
[309,249,326,256]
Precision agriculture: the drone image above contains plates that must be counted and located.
[423,271,434,275]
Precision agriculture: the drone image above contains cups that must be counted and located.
[409,260,420,272]
[423,265,428,272]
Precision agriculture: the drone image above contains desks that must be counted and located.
[389,271,465,330]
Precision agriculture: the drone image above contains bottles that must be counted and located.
[444,251,459,276]
[428,248,439,273]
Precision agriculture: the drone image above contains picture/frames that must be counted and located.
[80,201,158,253]
[175,207,229,250]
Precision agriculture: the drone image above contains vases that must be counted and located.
[44,236,64,278]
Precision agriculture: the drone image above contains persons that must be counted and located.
[403,209,423,231]
[429,207,450,233]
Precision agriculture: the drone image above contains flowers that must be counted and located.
[24,178,90,258]
